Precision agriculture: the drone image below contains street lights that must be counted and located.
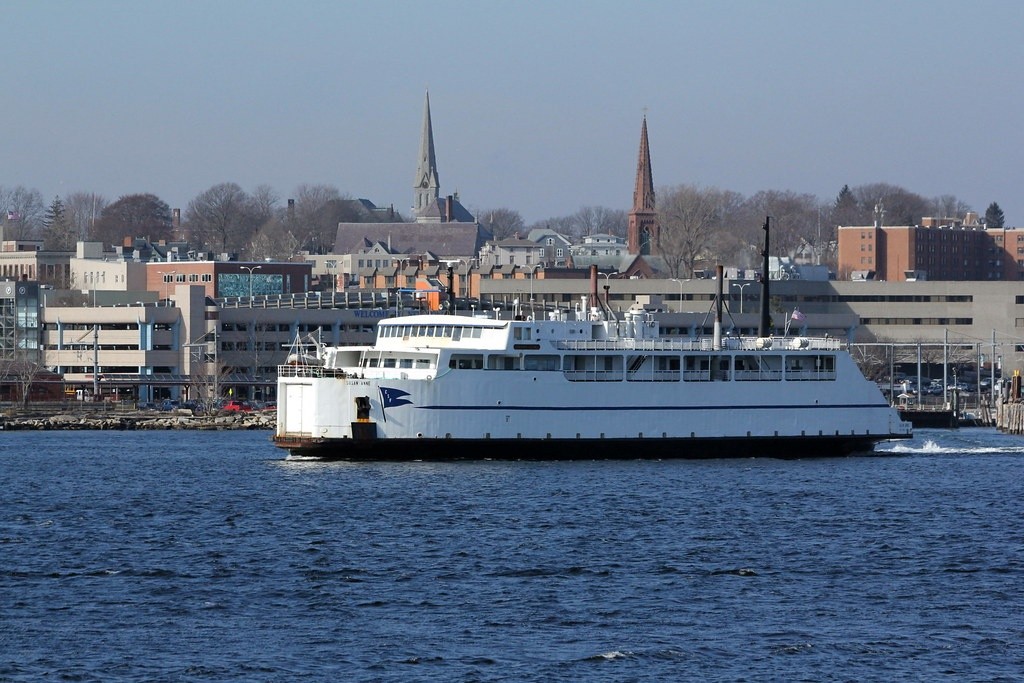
[240,266,263,307]
[522,265,541,299]
[733,283,751,314]
[158,271,175,307]
[84,273,105,308]
[458,258,475,297]
[673,279,691,313]
[393,258,411,293]
[325,262,343,292]
[598,271,617,285]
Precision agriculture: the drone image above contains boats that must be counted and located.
[273,216,914,459]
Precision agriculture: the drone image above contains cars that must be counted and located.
[135,399,276,412]
[930,379,944,387]
[927,385,944,394]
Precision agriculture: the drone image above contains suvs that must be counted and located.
[893,373,906,383]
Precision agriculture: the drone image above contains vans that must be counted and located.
[947,383,970,391]
[980,378,991,388]
